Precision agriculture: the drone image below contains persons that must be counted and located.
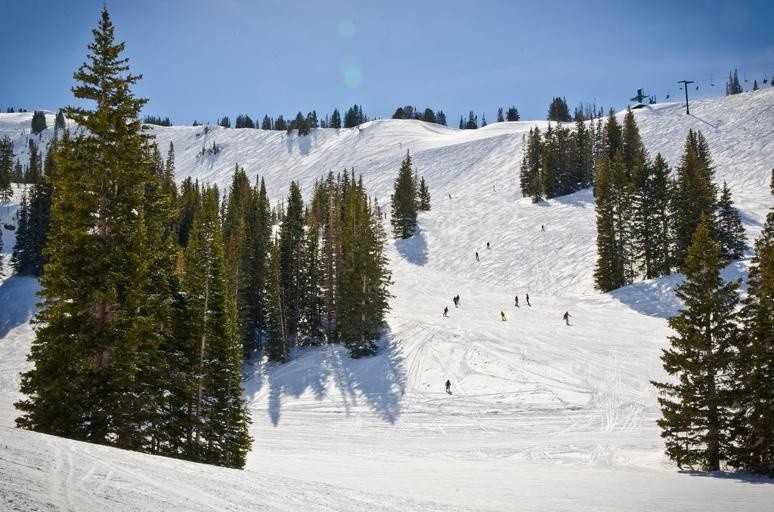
[563,311,571,326]
[526,294,530,305]
[445,380,451,392]
[514,296,519,307]
[500,311,506,321]
[443,307,449,317]
